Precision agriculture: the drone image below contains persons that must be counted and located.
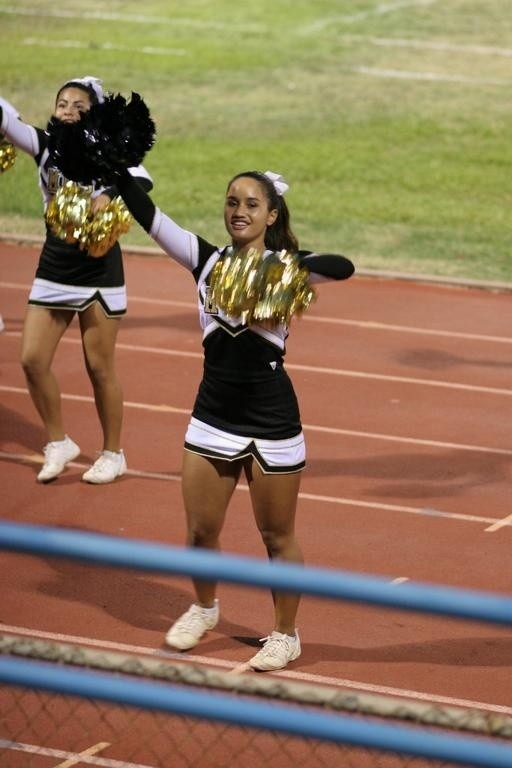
[87,148,355,672]
[0,74,152,486]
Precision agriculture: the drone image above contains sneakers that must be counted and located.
[165,598,220,650]
[82,448,127,485]
[247,627,302,671]
[36,433,81,482]
